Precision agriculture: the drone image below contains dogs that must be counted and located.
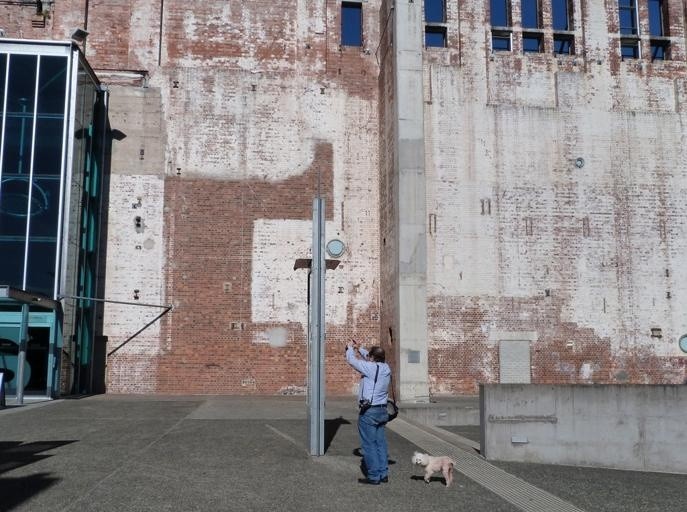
[410,451,455,488]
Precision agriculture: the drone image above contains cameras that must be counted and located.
[344,340,356,350]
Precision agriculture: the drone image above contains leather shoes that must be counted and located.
[359,476,388,484]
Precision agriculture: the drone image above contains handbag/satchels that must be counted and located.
[386,400,399,421]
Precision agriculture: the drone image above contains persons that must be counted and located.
[346,339,391,485]
